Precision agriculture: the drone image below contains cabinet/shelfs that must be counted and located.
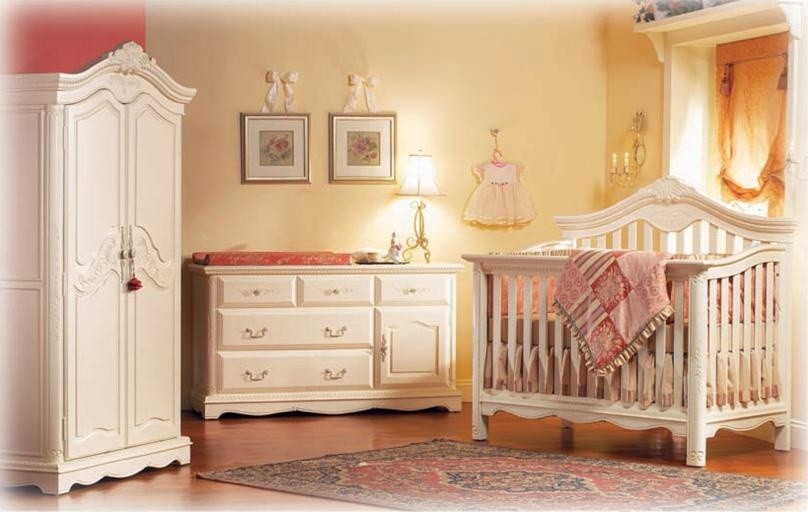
[185,262,466,424]
[1,38,197,495]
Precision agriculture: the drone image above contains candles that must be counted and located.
[612,152,629,170]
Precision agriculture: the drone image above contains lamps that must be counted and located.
[393,146,449,263]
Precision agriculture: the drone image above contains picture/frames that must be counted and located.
[239,111,398,188]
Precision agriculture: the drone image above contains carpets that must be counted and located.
[194,437,808,512]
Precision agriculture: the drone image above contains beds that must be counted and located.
[463,175,795,470]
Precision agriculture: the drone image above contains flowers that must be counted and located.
[631,1,717,24]
